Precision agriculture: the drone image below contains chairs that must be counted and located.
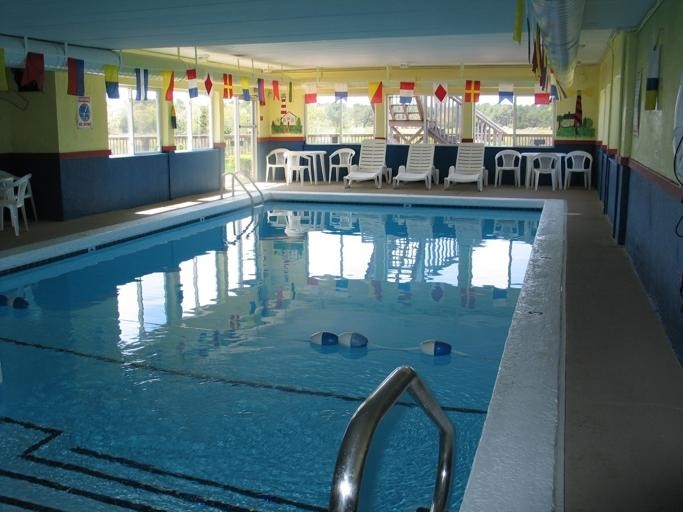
[267,148,289,182]
[0,169,41,237]
[494,149,522,188]
[343,140,392,189]
[328,148,355,185]
[444,143,489,192]
[284,152,313,184]
[564,151,593,189]
[393,143,440,190]
[530,154,558,191]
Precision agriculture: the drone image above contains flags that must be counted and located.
[398,81,414,103]
[20,52,44,92]
[367,80,382,103]
[159,70,174,101]
[134,67,148,101]
[512,0,568,104]
[202,72,213,96]
[66,57,84,95]
[304,83,317,104]
[222,72,232,99]
[334,81,347,102]
[239,76,250,101]
[257,78,265,104]
[0,48,9,91]
[104,64,120,98]
[464,80,480,102]
[498,81,513,104]
[433,79,449,104]
[185,68,198,98]
[272,79,280,101]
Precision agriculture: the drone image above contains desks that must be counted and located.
[520,151,567,190]
[284,151,326,184]
